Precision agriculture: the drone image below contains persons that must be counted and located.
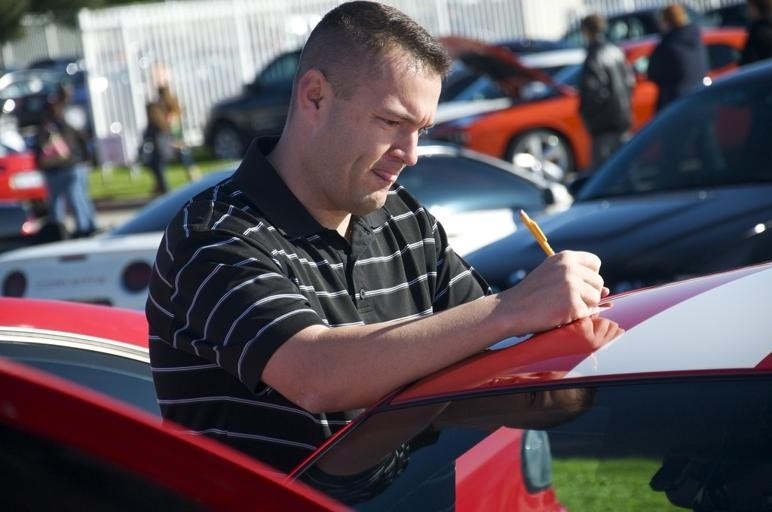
[645,3,728,171]
[29,94,103,238]
[133,102,172,196]
[575,13,638,186]
[146,346,594,506]
[153,79,200,182]
[145,0,612,415]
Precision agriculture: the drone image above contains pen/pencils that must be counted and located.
[519,209,555,257]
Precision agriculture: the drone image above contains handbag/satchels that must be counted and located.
[38,123,69,169]
